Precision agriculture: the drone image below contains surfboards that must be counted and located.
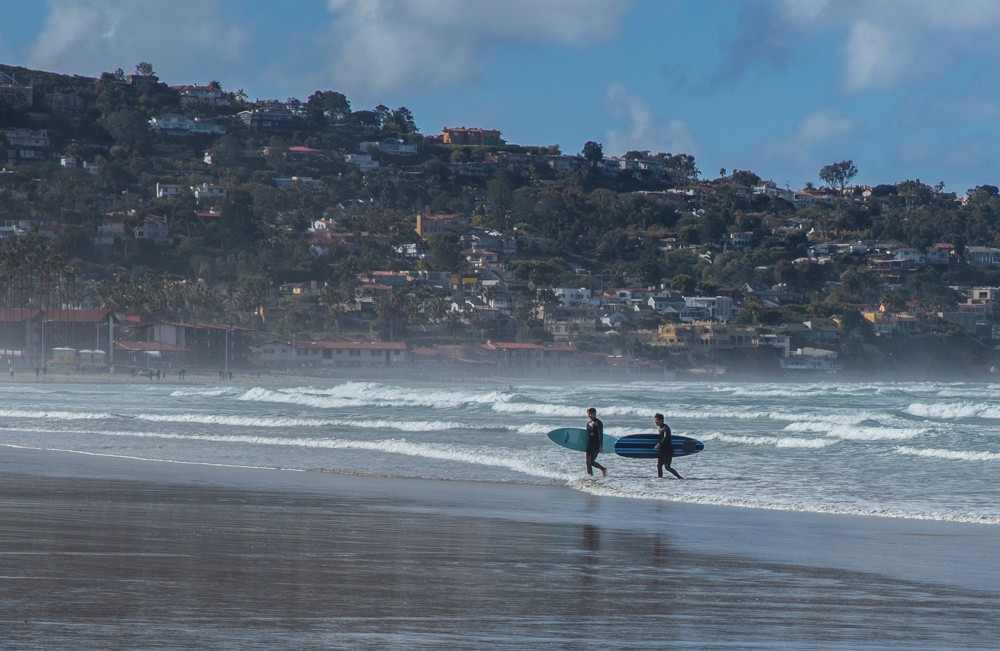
[613,434,704,459]
[547,427,619,453]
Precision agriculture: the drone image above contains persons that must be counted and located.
[82,366,85,376]
[586,408,606,476]
[219,370,232,380]
[36,367,40,376]
[156,370,160,380]
[42,367,46,376]
[149,371,153,380]
[179,370,185,380]
[654,413,683,479]
[10,368,14,376]
